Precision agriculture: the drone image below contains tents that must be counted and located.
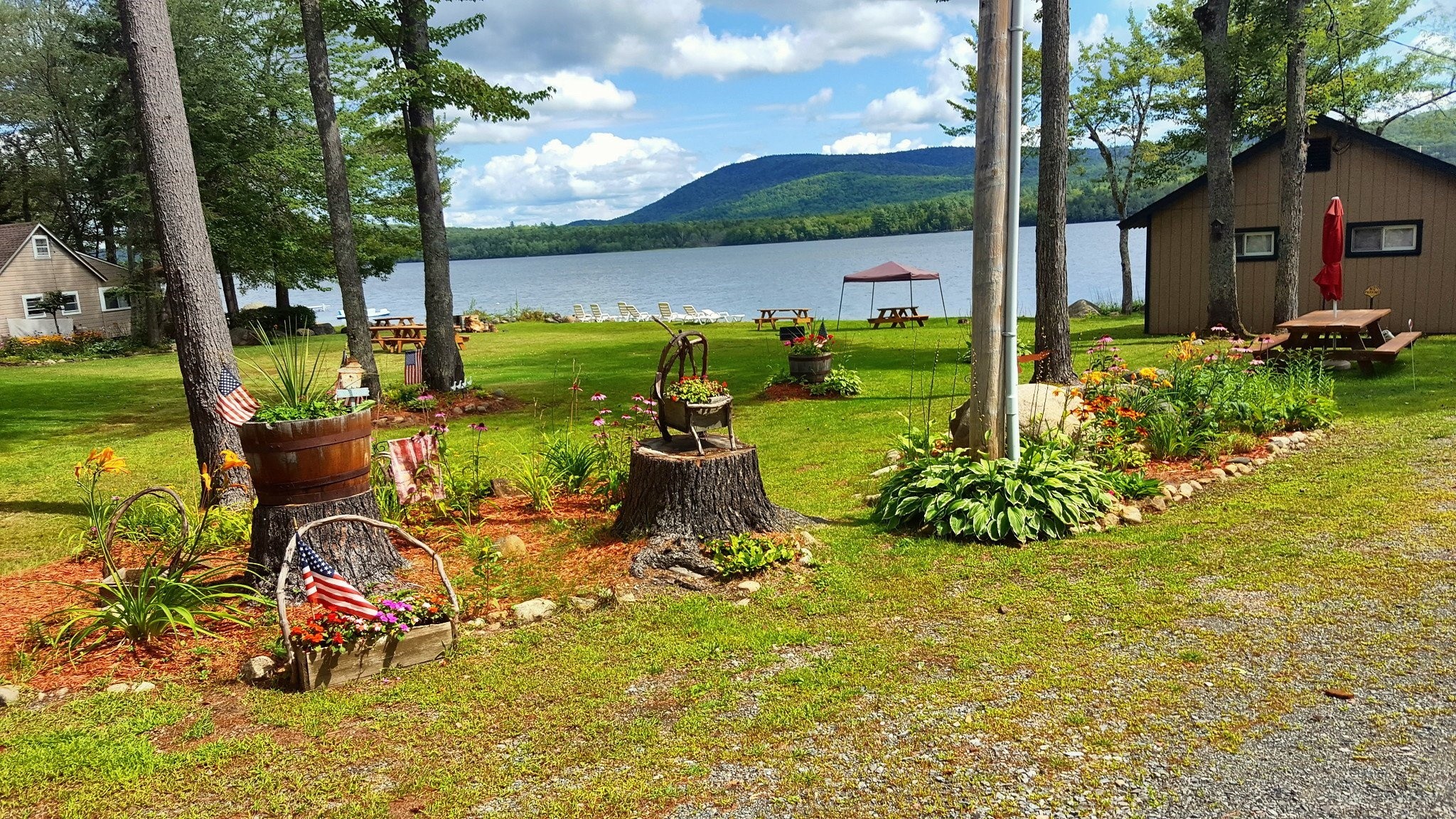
[836,261,948,331]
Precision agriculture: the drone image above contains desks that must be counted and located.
[373,317,415,326]
[368,324,465,353]
[757,308,811,331]
[873,306,925,330]
[1274,308,1395,379]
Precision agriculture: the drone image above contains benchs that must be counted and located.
[371,335,470,341]
[1248,333,1291,354]
[752,317,817,319]
[867,315,929,320]
[1374,331,1422,355]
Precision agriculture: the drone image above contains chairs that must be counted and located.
[572,301,746,325]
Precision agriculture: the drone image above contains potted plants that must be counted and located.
[228,311,377,505]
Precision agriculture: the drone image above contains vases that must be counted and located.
[788,351,832,382]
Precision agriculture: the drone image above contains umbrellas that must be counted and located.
[1312,196,1344,318]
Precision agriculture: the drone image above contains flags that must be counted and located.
[295,533,378,621]
[404,348,422,386]
[213,367,262,427]
[387,434,446,505]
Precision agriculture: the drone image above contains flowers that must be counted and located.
[289,591,455,656]
[664,375,730,402]
[778,309,842,356]
